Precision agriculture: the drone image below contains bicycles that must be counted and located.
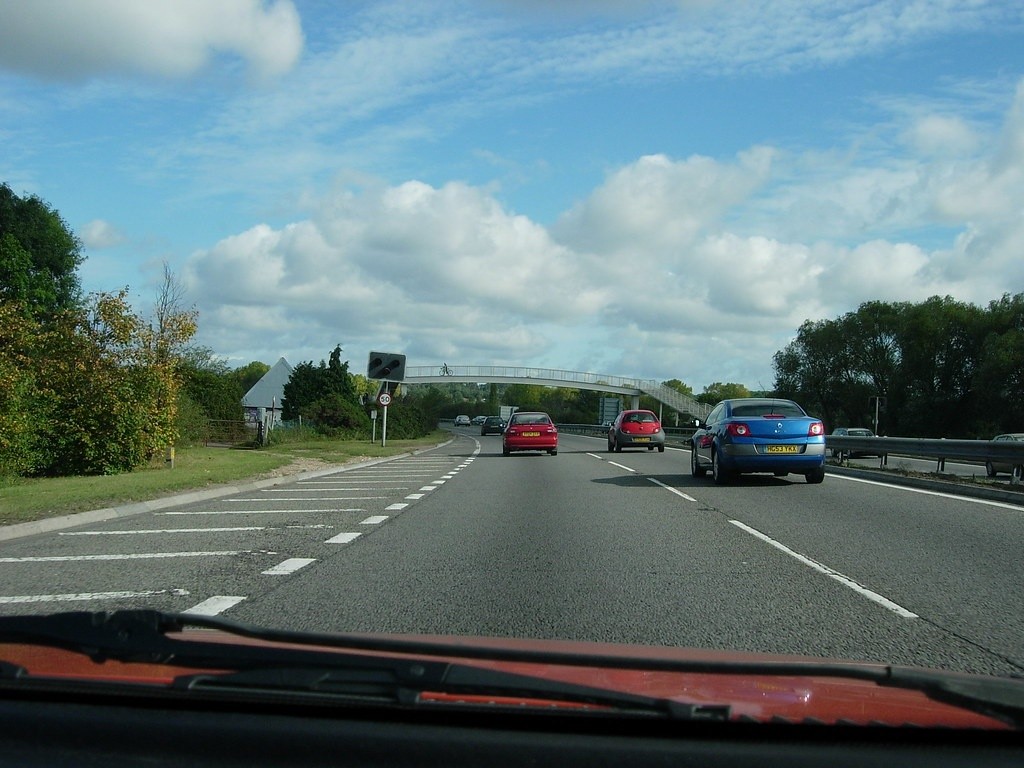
[439,368,453,376]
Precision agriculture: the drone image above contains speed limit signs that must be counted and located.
[378,393,393,406]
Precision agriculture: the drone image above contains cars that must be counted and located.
[607,410,666,453]
[830,428,882,459]
[473,416,487,426]
[481,417,507,436]
[688,397,826,486]
[454,414,471,427]
[499,412,558,457]
[985,433,1024,481]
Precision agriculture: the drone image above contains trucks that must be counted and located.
[498,406,519,426]
